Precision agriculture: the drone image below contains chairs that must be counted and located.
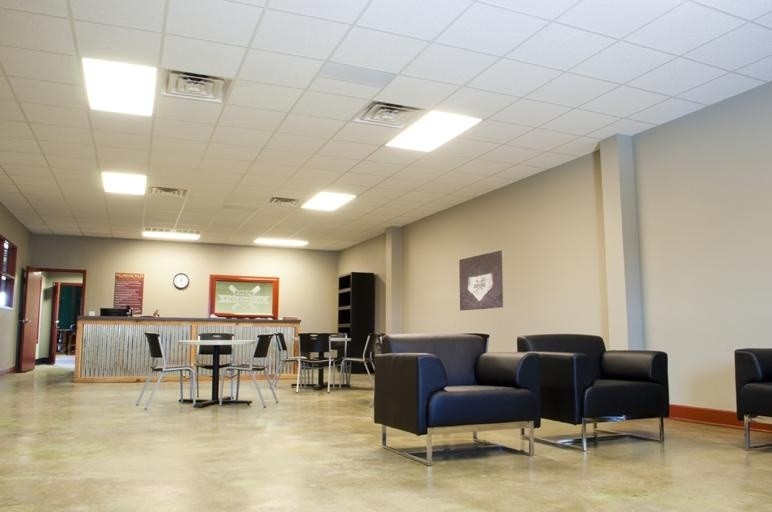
[374,335,543,466]
[274,332,375,393]
[518,334,669,451]
[219,334,278,408]
[197,332,235,398]
[734,348,772,448]
[67,332,76,354]
[137,334,196,410]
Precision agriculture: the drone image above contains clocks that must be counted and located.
[173,273,189,289]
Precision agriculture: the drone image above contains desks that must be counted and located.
[179,339,254,408]
[57,328,71,354]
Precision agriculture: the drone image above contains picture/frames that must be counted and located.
[208,274,280,318]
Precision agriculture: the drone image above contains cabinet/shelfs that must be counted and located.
[336,272,374,373]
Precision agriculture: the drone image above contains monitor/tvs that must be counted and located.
[101,309,132,316]
